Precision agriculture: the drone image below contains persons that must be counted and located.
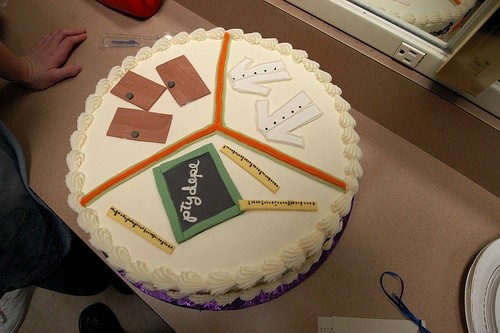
[0,27,136,298]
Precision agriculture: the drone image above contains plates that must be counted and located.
[464,238,499,333]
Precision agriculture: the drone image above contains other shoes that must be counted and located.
[76,301,129,333]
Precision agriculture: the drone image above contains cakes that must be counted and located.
[65,27,363,311]
[357,0,477,33]
